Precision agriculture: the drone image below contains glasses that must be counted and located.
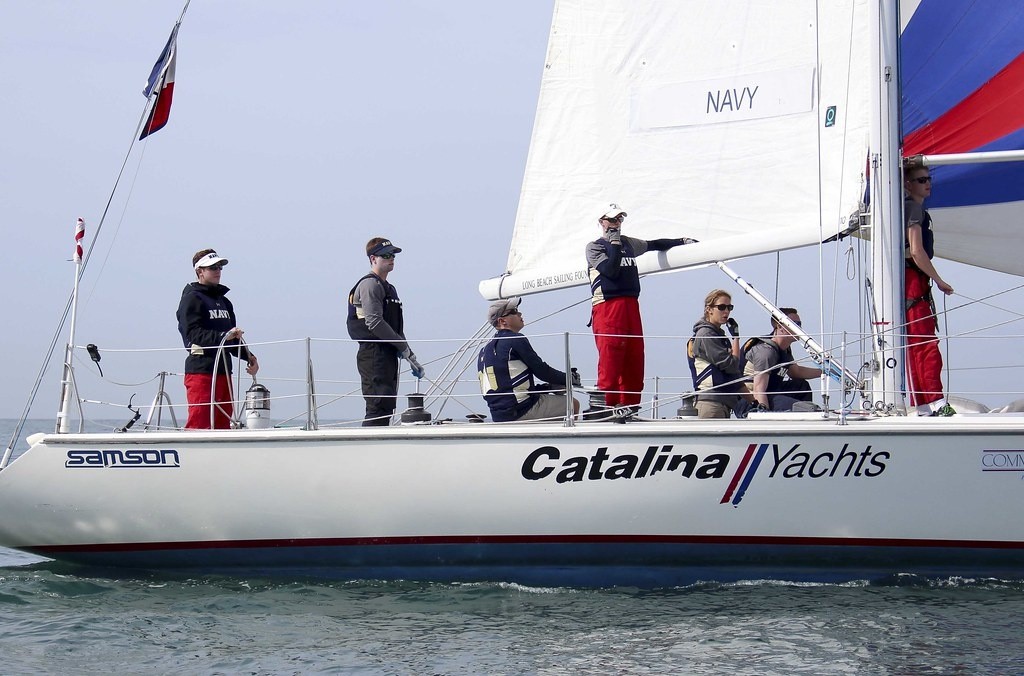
[206,264,222,270]
[778,321,802,327]
[910,177,932,184]
[380,251,396,259]
[602,216,624,223]
[505,307,519,316]
[708,304,733,311]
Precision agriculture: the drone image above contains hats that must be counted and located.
[195,253,228,268]
[367,240,402,257]
[596,201,627,220]
[487,297,522,326]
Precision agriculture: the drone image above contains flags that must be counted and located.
[138,21,180,141]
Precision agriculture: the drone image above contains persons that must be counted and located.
[740,307,838,412]
[176,249,258,430]
[477,297,584,420]
[901,164,958,416]
[346,238,426,426]
[586,202,699,419]
[688,289,760,419]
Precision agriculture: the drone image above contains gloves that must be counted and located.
[726,318,739,339]
[570,371,581,385]
[681,237,698,245]
[606,226,621,243]
[399,347,412,359]
[548,382,567,395]
[411,362,425,379]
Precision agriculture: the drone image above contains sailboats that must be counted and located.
[0,0,1024,575]
